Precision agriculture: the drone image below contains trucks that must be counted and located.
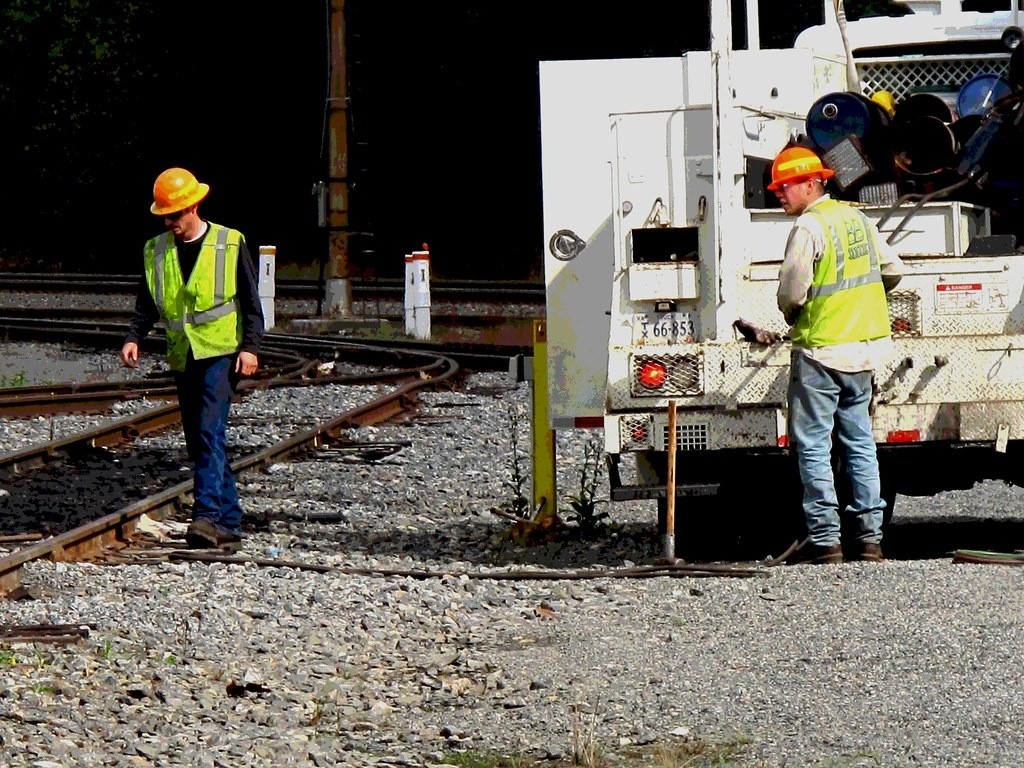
[539,0,1023,562]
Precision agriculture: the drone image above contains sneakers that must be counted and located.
[785,543,843,564]
[217,531,242,551]
[184,516,218,547]
[848,542,884,561]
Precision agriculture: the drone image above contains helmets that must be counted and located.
[766,147,836,191]
[149,166,210,216]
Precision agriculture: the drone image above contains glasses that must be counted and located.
[161,210,182,220]
[774,180,805,191]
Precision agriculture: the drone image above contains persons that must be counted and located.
[767,147,903,562]
[119,168,265,552]
